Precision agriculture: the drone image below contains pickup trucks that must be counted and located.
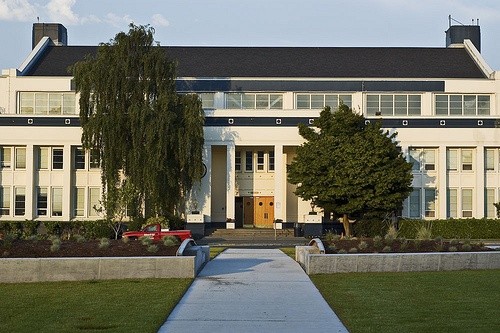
[122,222,193,242]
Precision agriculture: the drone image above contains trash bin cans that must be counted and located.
[293,223,302,237]
